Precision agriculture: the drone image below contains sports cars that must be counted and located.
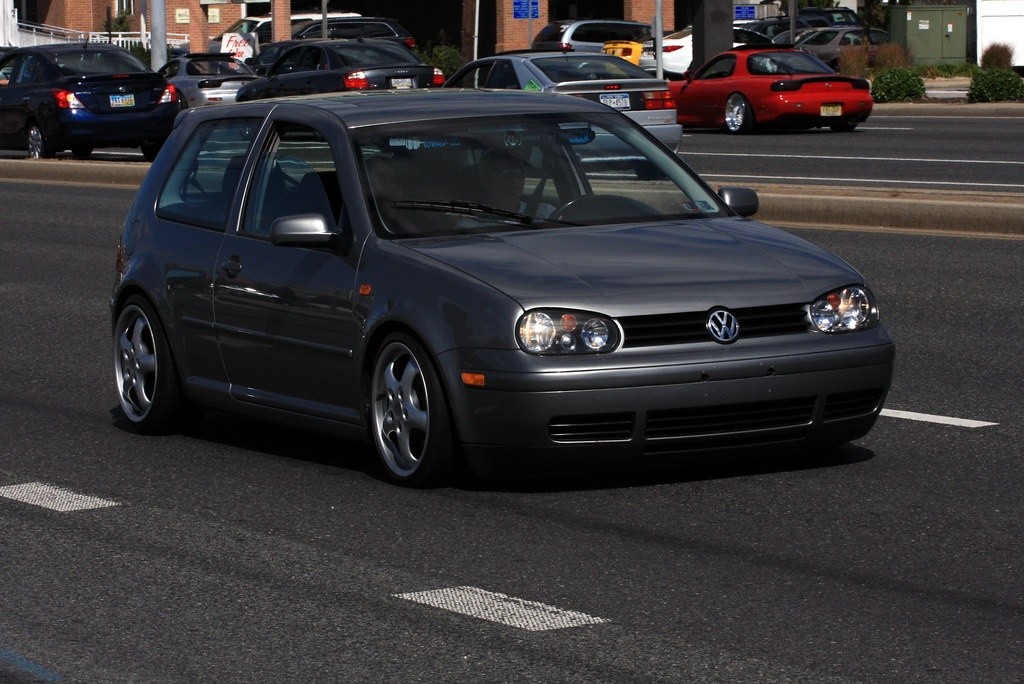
[671,47,875,133]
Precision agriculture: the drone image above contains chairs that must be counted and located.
[219,67,238,75]
[208,155,288,231]
[293,170,350,233]
[176,62,200,77]
[432,164,482,233]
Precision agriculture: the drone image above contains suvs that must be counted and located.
[260,16,418,58]
[532,18,653,80]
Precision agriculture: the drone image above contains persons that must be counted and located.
[453,148,563,228]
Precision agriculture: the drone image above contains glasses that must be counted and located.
[493,168,527,179]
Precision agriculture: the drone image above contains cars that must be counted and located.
[733,7,891,71]
[180,12,363,71]
[236,40,444,140]
[432,52,684,180]
[0,43,182,161]
[638,20,776,79]
[109,85,896,483]
[602,29,674,68]
[156,53,261,110]
